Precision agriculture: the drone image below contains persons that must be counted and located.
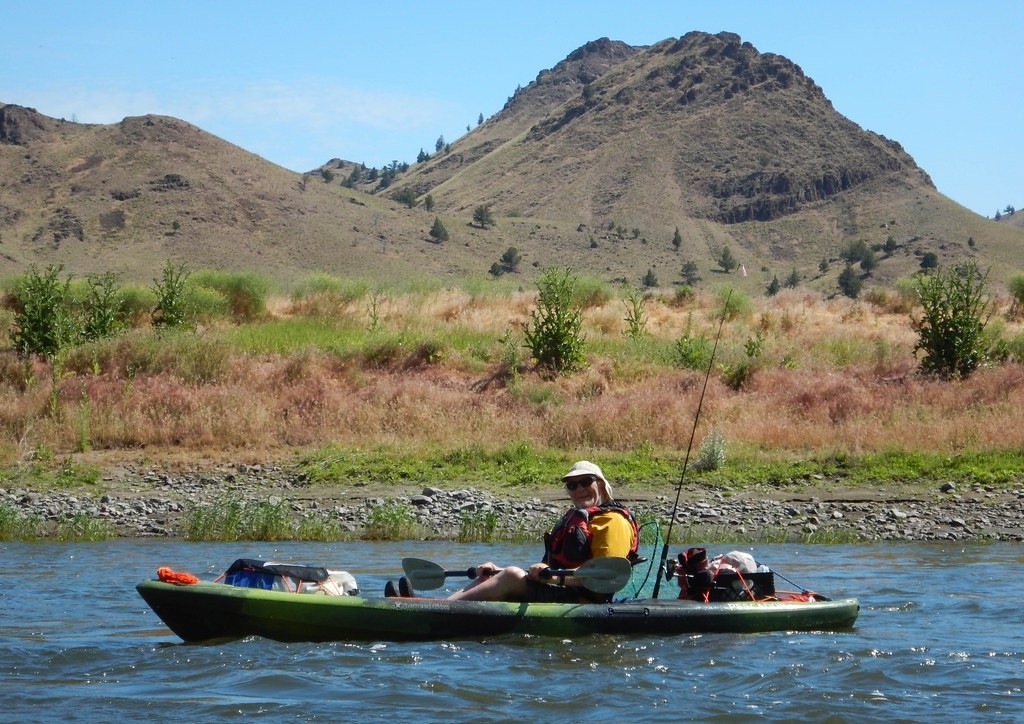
[383,461,637,606]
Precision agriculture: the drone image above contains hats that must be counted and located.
[561,460,614,501]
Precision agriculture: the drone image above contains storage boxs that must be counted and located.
[687,569,775,603]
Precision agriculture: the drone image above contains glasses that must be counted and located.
[566,476,598,490]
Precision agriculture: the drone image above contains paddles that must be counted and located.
[402,558,631,593]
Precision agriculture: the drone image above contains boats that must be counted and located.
[136,577,861,642]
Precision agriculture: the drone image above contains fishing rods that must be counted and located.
[652,263,748,602]
